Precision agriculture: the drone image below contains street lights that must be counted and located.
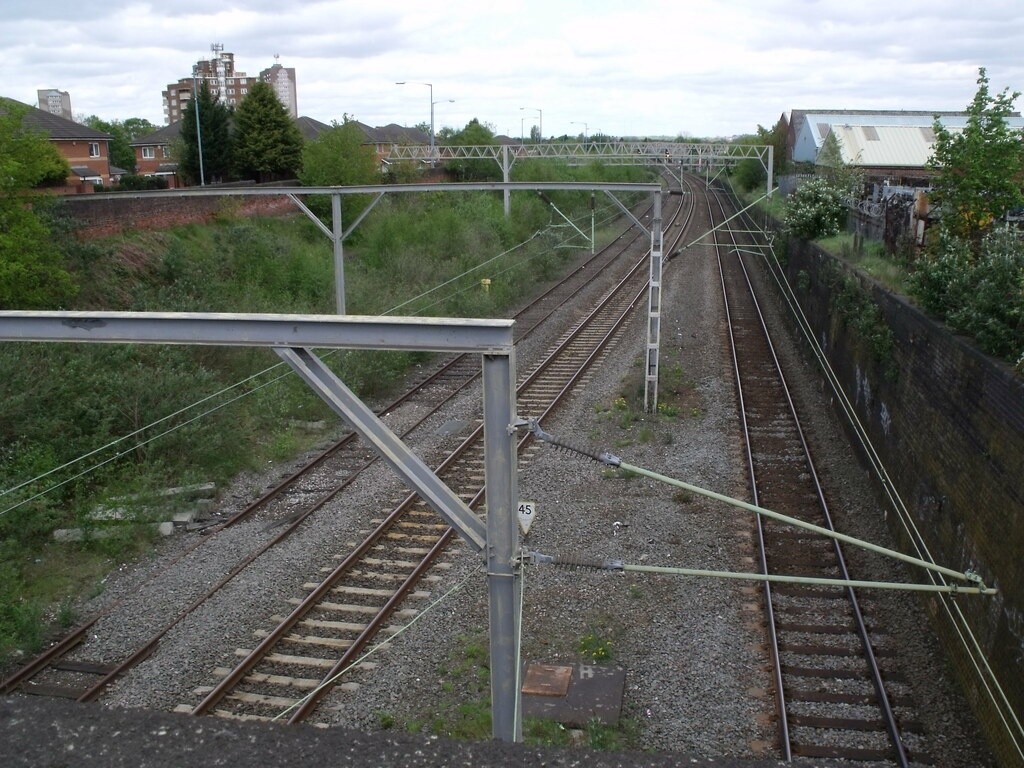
[520,107,542,143]
[395,81,456,169]
[192,64,206,187]
[570,121,588,151]
